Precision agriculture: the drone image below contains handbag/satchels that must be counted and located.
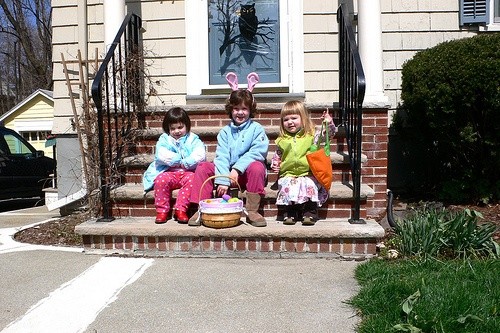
[307,124,332,191]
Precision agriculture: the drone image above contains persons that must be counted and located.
[188,89,269,227]
[271,100,336,225]
[144,107,206,223]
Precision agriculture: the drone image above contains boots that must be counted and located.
[245,191,267,227]
[188,209,202,226]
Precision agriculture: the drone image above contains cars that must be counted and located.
[0,123,57,204]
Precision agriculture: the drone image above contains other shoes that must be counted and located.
[155,212,171,222]
[283,208,297,224]
[173,209,188,223]
[301,204,319,223]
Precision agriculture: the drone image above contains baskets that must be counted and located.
[199,175,244,228]
